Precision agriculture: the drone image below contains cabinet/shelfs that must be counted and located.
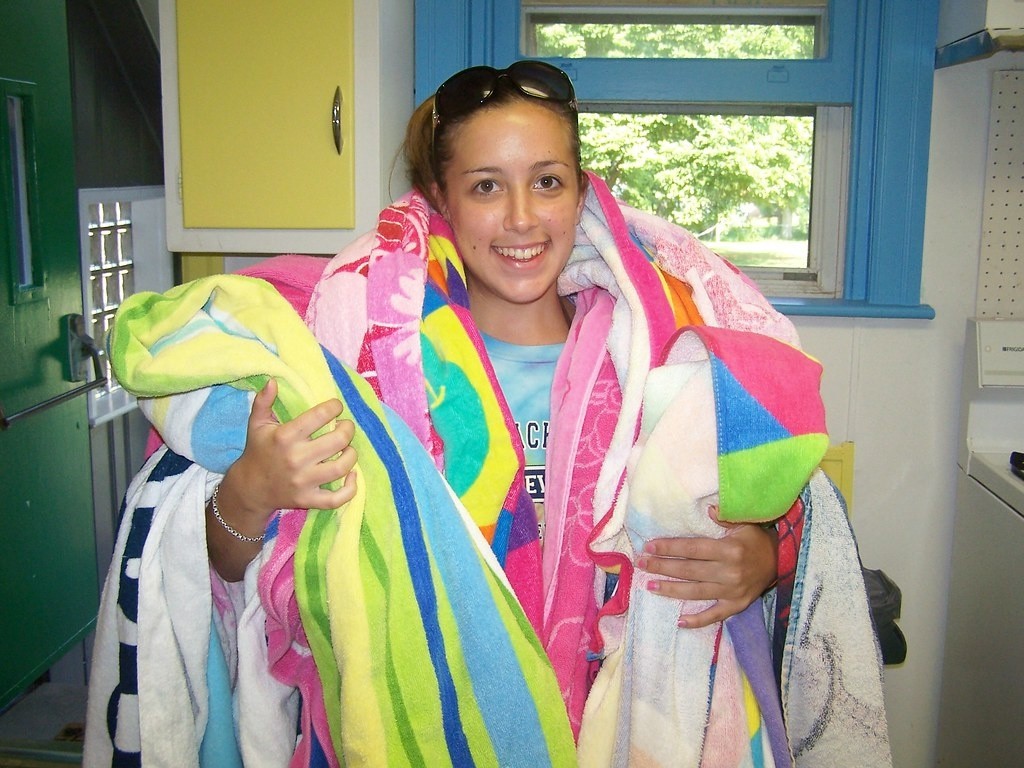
[159,0,415,254]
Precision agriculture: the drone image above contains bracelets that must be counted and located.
[212,483,267,543]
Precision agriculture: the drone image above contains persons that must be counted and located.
[202,60,853,768]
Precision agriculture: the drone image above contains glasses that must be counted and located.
[431,60,579,166]
